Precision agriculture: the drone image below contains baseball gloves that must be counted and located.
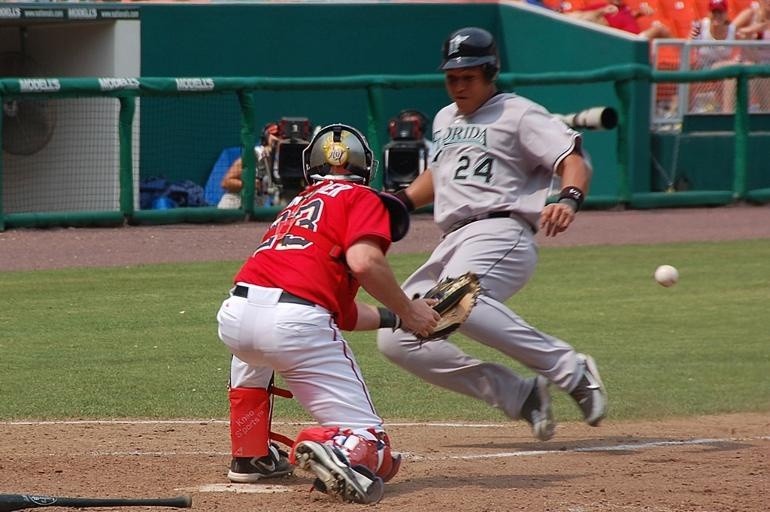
[410,271,481,346]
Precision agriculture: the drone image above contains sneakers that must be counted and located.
[291,439,375,506]
[228,444,293,483]
[570,353,608,427]
[520,375,555,442]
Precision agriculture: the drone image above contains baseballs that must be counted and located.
[654,266,679,288]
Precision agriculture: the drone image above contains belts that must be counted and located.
[489,209,510,218]
[232,284,311,307]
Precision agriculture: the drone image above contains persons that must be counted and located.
[378,110,438,195]
[214,119,292,212]
[560,1,770,133]
[373,24,609,442]
[214,122,488,506]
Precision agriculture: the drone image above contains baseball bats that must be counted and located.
[2,494,194,512]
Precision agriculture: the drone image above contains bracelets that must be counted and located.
[378,307,401,332]
[392,189,417,215]
[556,185,584,213]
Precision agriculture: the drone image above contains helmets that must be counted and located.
[436,26,500,83]
[302,123,379,187]
